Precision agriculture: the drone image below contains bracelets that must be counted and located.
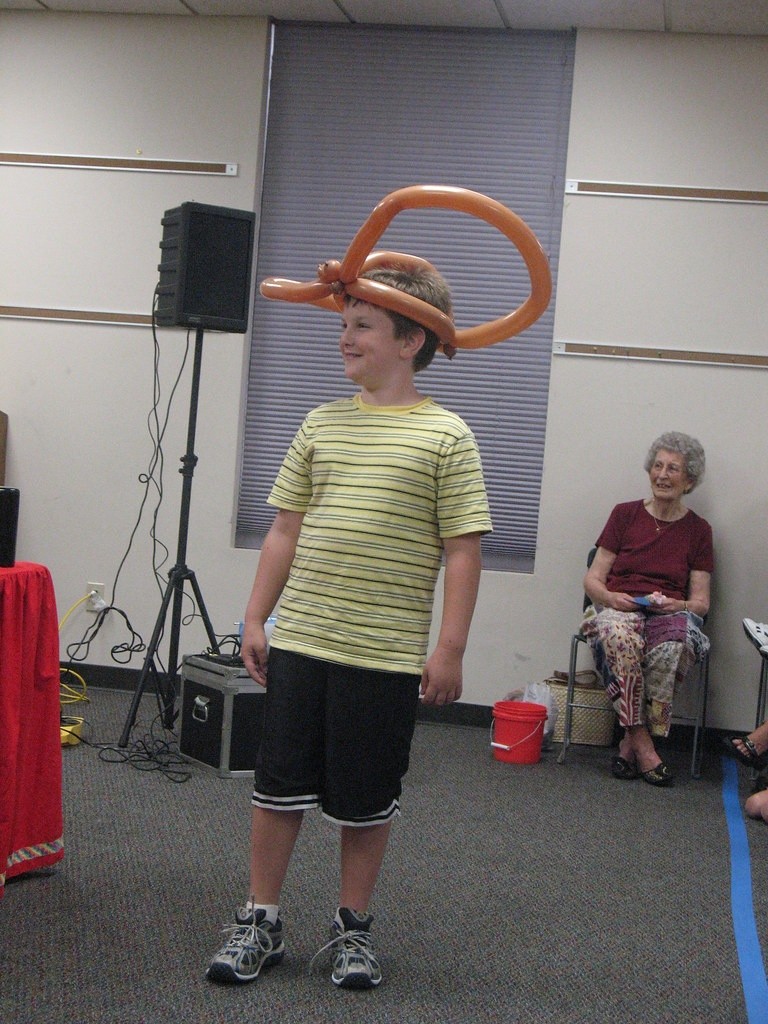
[683,600,689,612]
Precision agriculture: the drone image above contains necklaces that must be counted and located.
[653,500,678,531]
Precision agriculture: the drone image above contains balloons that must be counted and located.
[257,181,551,349]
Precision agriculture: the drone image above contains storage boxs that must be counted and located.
[176,655,268,780]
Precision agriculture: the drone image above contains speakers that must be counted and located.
[153,202,256,334]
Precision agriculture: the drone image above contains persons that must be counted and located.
[200,252,493,994]
[722,717,768,820]
[581,430,715,786]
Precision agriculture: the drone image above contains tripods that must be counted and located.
[118,329,220,749]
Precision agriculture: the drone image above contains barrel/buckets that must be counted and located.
[488,700,548,765]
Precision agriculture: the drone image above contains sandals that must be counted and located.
[724,734,765,771]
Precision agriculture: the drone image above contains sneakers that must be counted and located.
[206,896,286,987]
[308,905,382,989]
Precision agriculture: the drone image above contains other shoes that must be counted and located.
[612,741,639,780]
[635,753,673,787]
[743,617,768,659]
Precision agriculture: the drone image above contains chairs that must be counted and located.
[556,548,709,779]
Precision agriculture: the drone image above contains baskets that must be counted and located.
[547,682,616,746]
